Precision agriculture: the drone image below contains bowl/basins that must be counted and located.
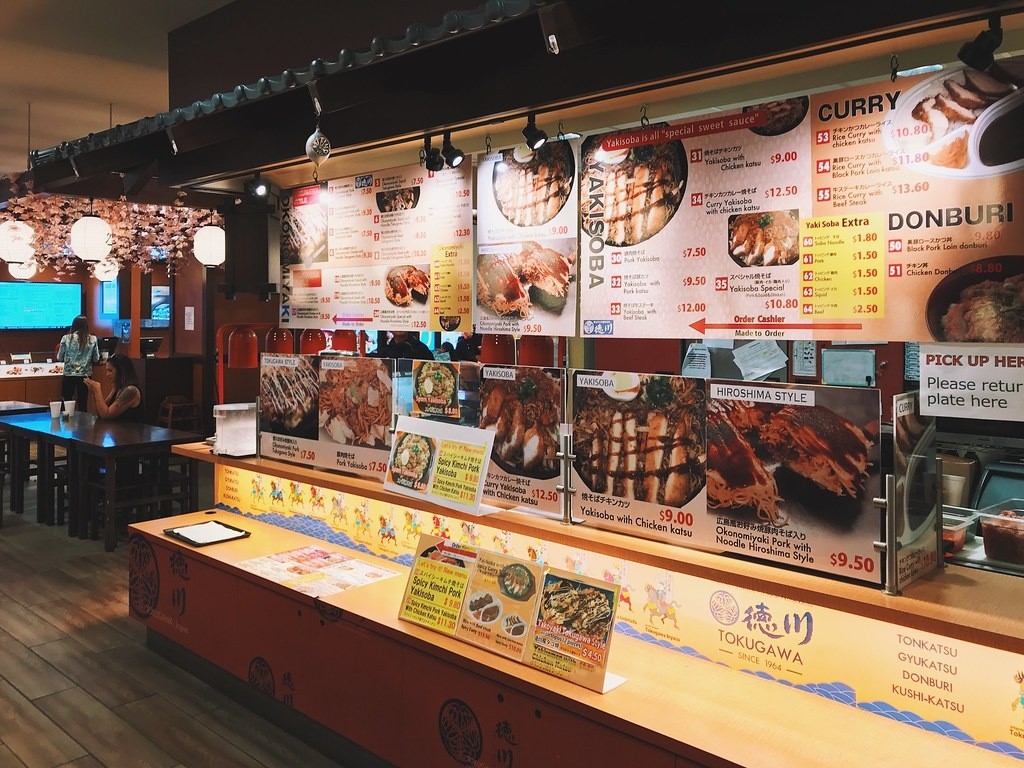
[413,362,458,414]
[389,433,434,488]
[497,564,536,602]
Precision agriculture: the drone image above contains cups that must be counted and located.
[50,402,62,418]
[101,352,109,363]
[62,411,69,420]
[64,401,76,418]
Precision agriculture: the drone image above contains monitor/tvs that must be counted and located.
[99,336,118,360]
[0,281,83,331]
[141,337,163,358]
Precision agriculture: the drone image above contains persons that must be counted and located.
[54,316,100,411]
[386,327,482,361]
[83,353,144,424]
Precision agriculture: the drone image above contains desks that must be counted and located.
[0,400,49,415]
[0,411,204,554]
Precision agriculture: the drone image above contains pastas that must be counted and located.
[319,356,393,445]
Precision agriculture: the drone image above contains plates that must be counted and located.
[728,209,799,268]
[480,364,561,480]
[501,614,527,638]
[925,256,1024,344]
[492,140,575,227]
[439,316,461,332]
[742,96,809,136]
[467,591,503,624]
[376,186,420,213]
[581,122,688,247]
[881,62,1024,179]
[573,392,706,508]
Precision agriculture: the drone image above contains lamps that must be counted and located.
[520,113,548,151]
[957,14,1003,72]
[442,130,465,169]
[250,172,266,196]
[423,136,444,172]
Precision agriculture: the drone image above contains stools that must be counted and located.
[158,403,200,432]
